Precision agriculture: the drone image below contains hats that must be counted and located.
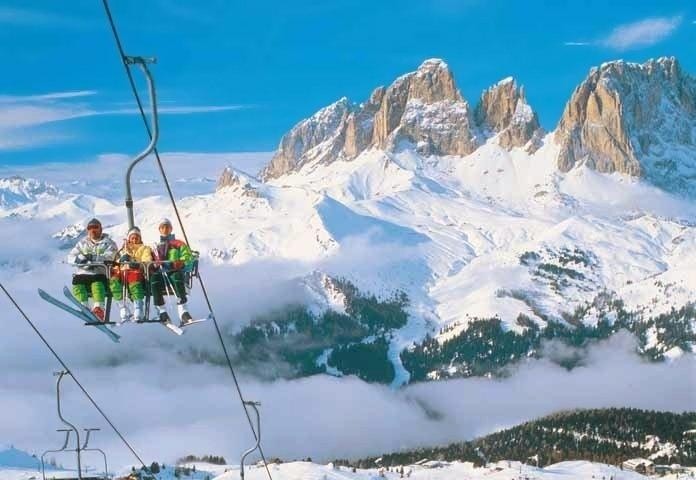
[158,219,172,233]
[127,226,141,242]
[76,253,92,264]
[88,219,101,225]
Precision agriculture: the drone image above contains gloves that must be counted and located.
[174,260,183,268]
[130,262,140,267]
[120,254,129,263]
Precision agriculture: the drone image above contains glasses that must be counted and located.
[87,226,100,230]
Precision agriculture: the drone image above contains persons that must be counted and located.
[108,226,153,321]
[150,219,193,324]
[67,218,118,320]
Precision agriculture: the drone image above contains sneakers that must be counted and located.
[181,311,191,324]
[134,309,143,321]
[158,311,170,324]
[93,307,105,320]
[120,307,132,318]
